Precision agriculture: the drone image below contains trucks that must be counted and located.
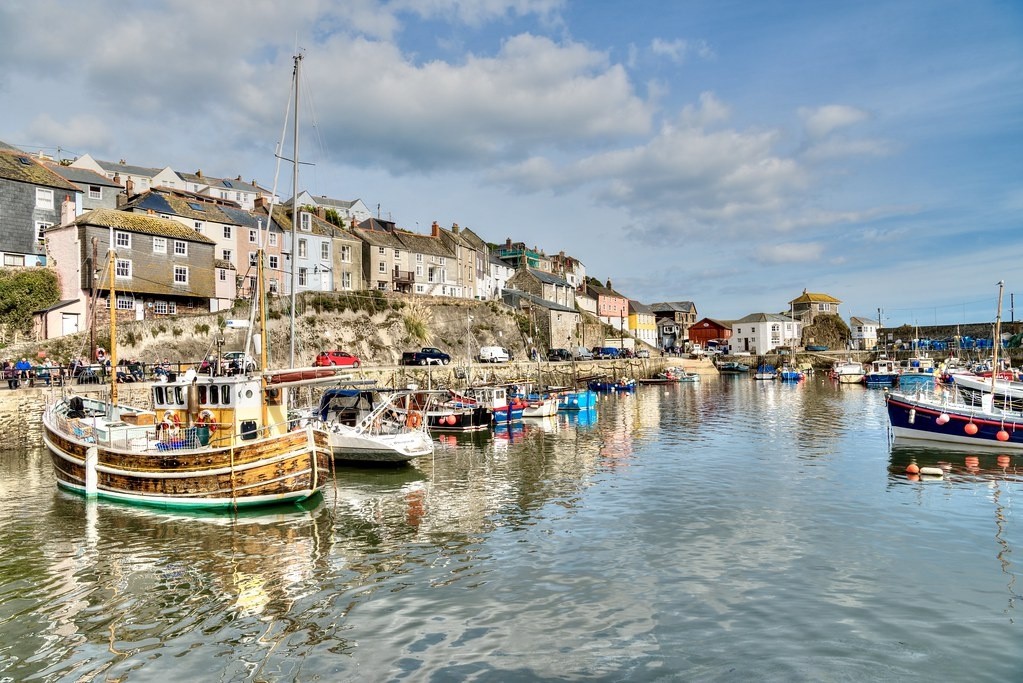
[479,346,510,364]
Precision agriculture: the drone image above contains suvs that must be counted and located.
[619,348,633,358]
[592,347,620,359]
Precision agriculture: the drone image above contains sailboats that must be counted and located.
[884,279,1023,449]
[780,301,806,381]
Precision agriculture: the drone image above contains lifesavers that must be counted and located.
[197,411,217,439]
[162,410,182,436]
[96,348,107,361]
[408,413,421,428]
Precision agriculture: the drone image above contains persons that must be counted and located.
[661,349,665,357]
[619,348,632,358]
[203,358,210,371]
[674,347,682,357]
[44,358,66,386]
[0,357,31,389]
[70,345,171,384]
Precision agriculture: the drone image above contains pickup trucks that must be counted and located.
[402,348,451,365]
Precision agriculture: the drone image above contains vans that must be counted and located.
[572,346,594,361]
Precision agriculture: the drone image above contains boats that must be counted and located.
[286,379,451,471]
[46,250,336,512]
[828,308,1023,401]
[387,294,700,436]
[753,353,779,380]
[884,437,1023,481]
[715,356,751,374]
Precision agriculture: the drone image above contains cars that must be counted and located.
[637,350,650,359]
[546,348,572,361]
[316,350,361,368]
[214,352,257,372]
[692,346,725,357]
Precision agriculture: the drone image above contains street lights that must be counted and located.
[1008,293,1016,337]
[680,312,696,356]
[877,308,890,344]
